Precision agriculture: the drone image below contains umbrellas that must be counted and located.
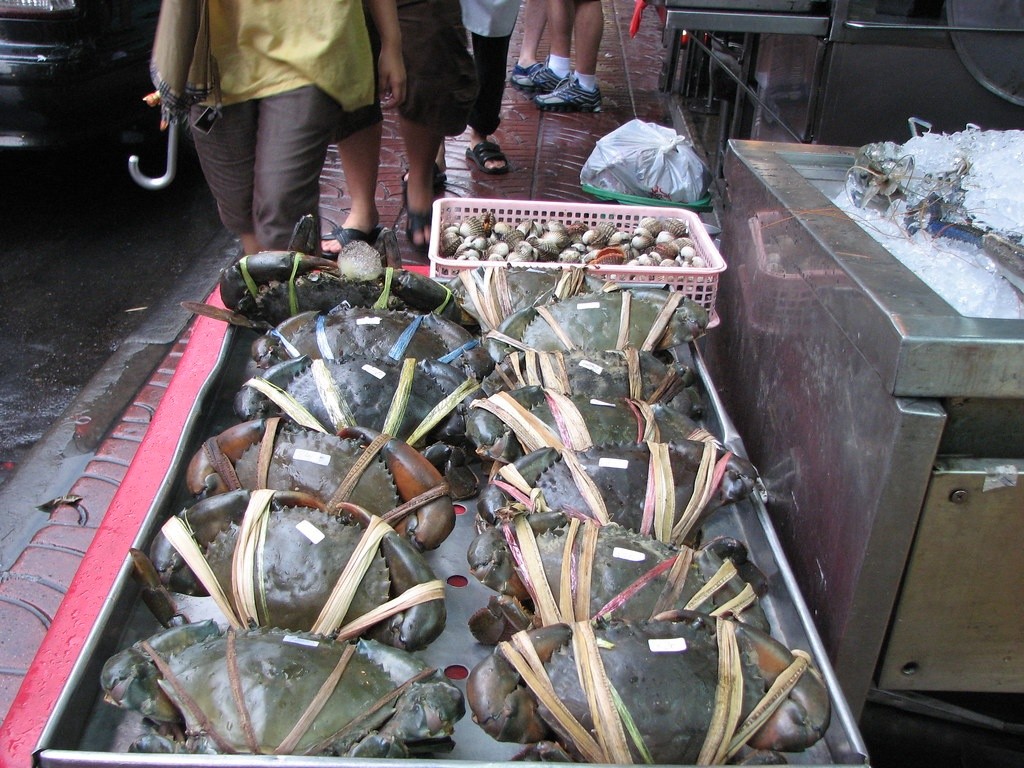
[129,0,215,190]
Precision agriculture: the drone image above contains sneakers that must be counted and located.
[510,55,572,94]
[533,71,602,113]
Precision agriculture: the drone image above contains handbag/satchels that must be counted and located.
[580,119,715,202]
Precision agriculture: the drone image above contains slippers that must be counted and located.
[400,161,447,190]
[320,224,385,260]
[466,142,509,174]
[403,181,433,252]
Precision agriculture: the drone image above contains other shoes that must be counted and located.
[512,62,544,75]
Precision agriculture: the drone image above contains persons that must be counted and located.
[154,0,408,257]
[318,0,455,257]
[511,0,605,109]
[401,0,521,189]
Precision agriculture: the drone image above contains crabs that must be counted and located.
[98,245,832,766]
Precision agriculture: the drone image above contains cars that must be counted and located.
[1,0,169,215]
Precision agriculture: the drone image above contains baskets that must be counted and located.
[582,184,714,216]
[427,196,727,330]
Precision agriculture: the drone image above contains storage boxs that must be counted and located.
[748,211,855,327]
[427,196,729,322]
